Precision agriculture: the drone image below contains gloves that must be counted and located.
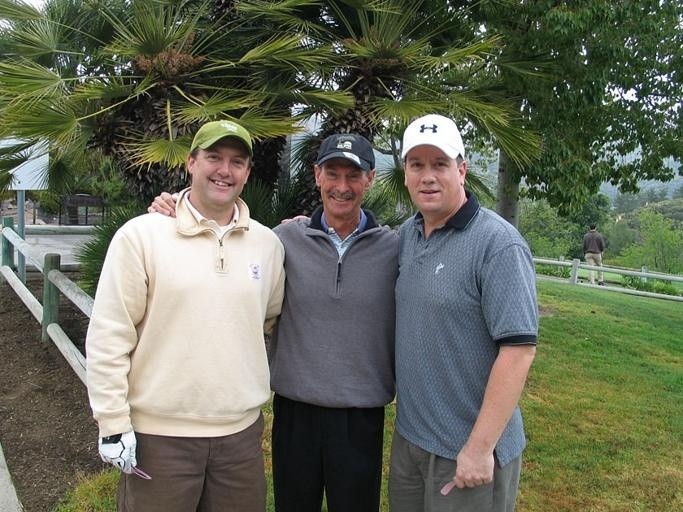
[97,429,137,475]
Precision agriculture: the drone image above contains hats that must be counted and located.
[316,134,375,172]
[187,120,253,159]
[401,114,465,161]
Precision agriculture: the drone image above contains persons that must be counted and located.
[581,223,606,287]
[80,117,286,512]
[279,110,541,512]
[143,131,402,512]
